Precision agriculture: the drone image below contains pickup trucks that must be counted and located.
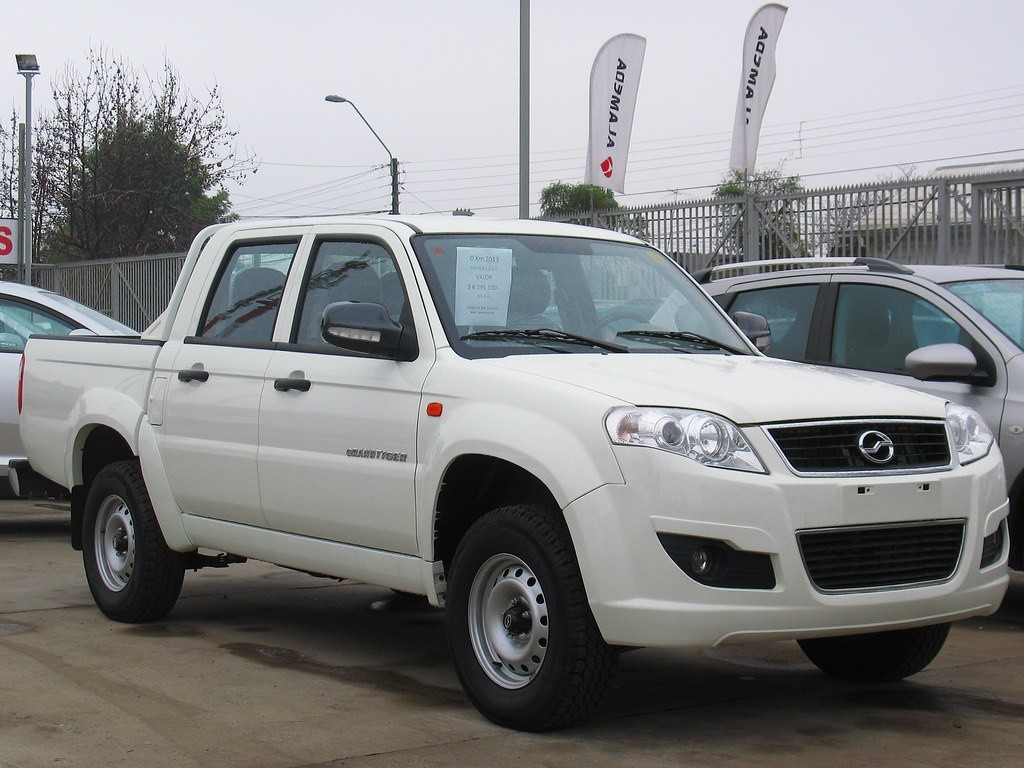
[13,211,1013,733]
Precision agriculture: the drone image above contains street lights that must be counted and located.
[326,94,401,215]
[14,52,42,286]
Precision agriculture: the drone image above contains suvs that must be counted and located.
[646,255,1023,579]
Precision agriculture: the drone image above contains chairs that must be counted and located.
[845,312,893,372]
[231,261,402,346]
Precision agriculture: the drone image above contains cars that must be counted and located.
[0,279,141,503]
